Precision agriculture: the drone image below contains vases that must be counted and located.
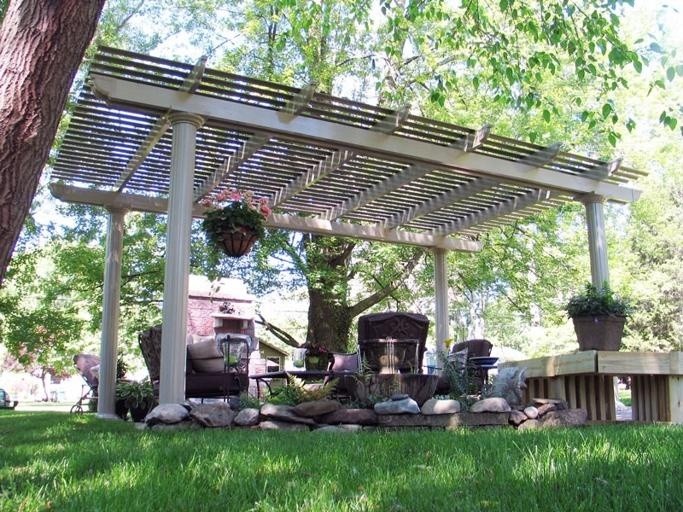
[211,227,259,257]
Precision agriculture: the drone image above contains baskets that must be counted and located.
[222,227,258,257]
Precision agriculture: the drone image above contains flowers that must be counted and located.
[199,188,272,239]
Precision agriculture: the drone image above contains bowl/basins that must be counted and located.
[469,356,498,366]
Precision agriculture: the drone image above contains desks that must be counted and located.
[500,352,681,425]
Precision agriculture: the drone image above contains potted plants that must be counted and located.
[116,376,154,420]
[564,278,631,352]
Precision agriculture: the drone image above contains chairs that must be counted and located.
[427,338,498,395]
[137,324,249,403]
[71,353,135,417]
[357,311,430,377]
[325,351,358,393]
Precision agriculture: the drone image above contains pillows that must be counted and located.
[187,338,233,373]
